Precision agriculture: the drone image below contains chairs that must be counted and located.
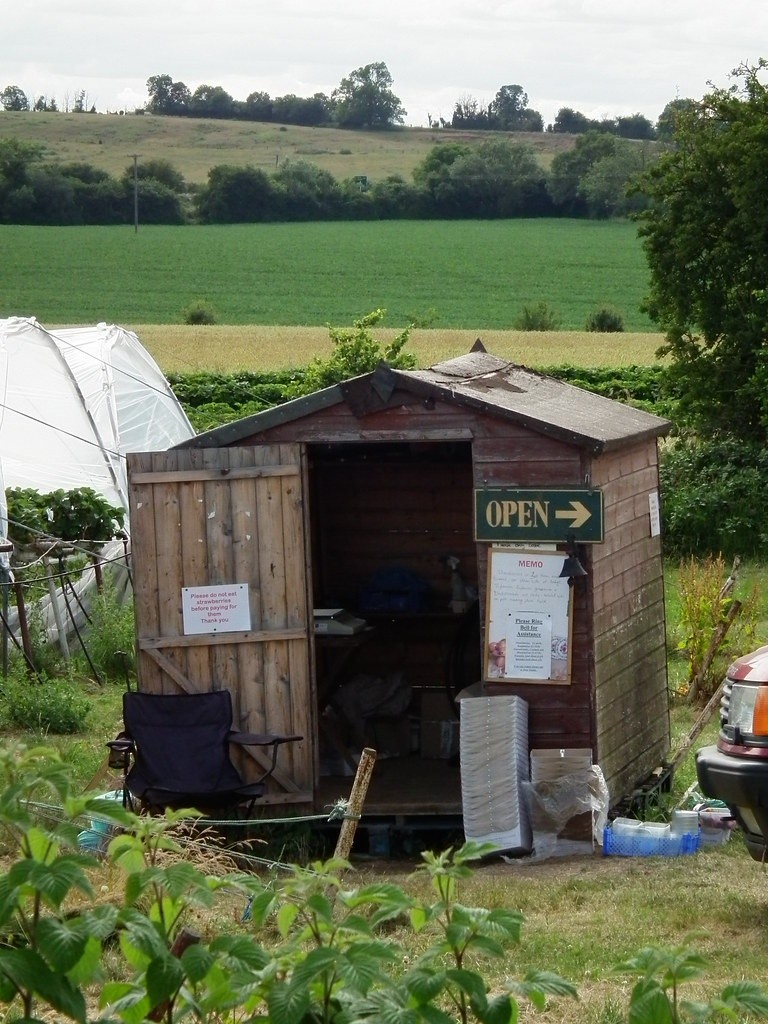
[106,690,304,868]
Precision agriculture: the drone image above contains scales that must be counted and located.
[314,608,367,636]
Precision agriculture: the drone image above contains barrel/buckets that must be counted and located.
[693,799,730,846]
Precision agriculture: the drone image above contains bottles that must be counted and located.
[451,560,465,601]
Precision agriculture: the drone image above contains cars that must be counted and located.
[694,646,768,864]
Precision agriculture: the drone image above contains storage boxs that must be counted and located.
[530,749,596,855]
[604,807,737,857]
[422,692,460,758]
[460,695,532,857]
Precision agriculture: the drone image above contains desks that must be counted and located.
[318,624,380,756]
[367,610,480,752]
